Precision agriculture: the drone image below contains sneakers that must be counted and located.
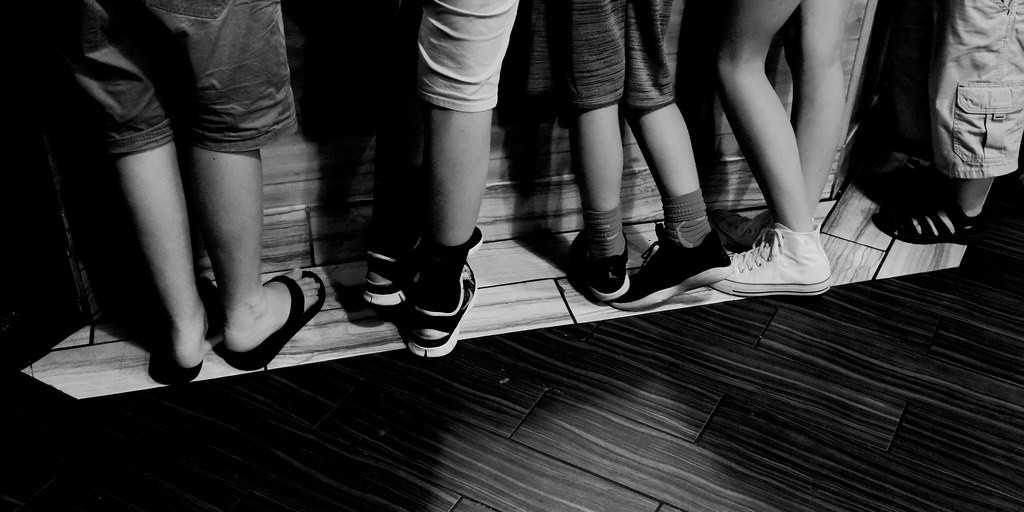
[364,225,482,306]
[569,227,630,302]
[714,206,774,254]
[405,260,478,357]
[709,221,831,297]
[608,222,735,312]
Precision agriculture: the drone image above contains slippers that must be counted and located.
[145,275,219,386]
[221,268,327,371]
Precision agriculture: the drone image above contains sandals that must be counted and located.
[875,202,990,247]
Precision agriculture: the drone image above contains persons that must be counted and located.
[64,0,521,384]
[548,0,1024,312]
